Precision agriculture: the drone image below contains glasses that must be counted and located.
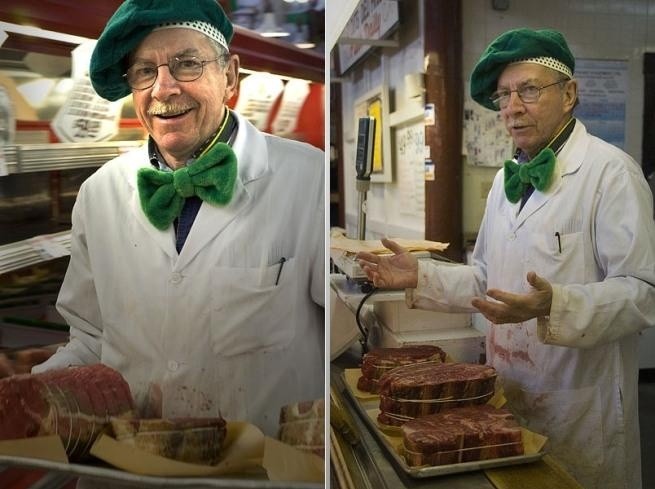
[489,80,568,110]
[121,55,221,91]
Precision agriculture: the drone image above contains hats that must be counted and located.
[87,0,236,102]
[467,28,576,111]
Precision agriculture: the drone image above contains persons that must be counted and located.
[27,1,325,439]
[354,27,654,489]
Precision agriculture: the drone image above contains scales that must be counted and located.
[335,112,434,293]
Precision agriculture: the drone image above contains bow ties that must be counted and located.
[503,147,558,205]
[136,142,240,231]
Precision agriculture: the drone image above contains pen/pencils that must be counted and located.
[555,232,562,254]
[275,257,286,286]
